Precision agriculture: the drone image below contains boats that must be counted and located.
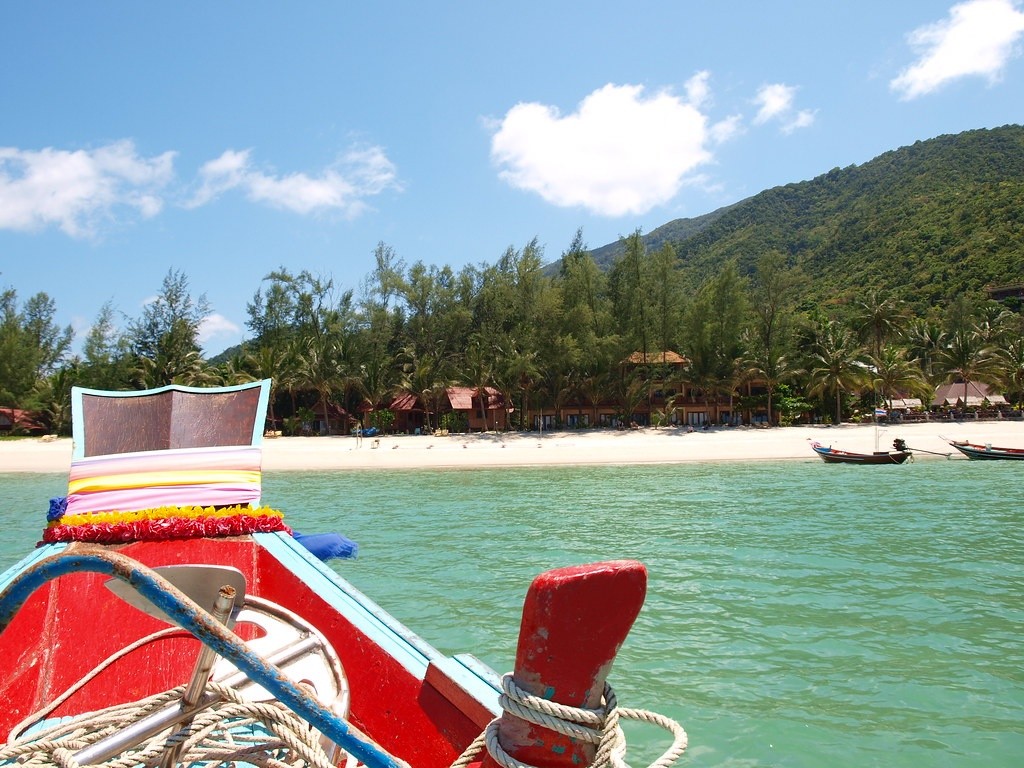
[806,437,914,465]
[939,433,1024,461]
